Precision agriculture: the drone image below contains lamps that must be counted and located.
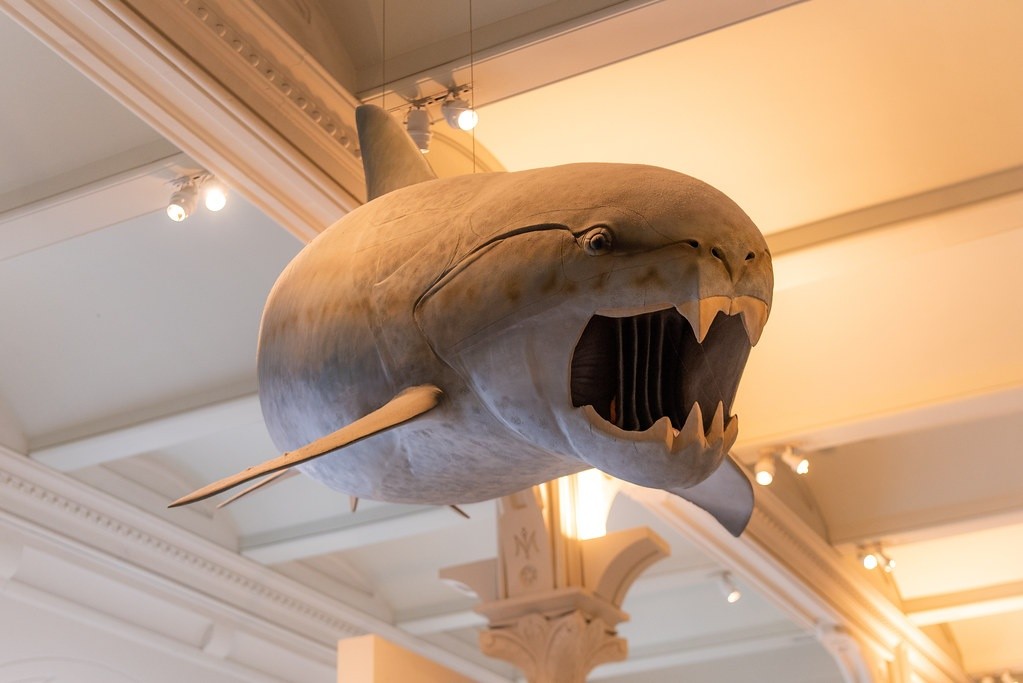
[196,172,229,213]
[438,80,479,132]
[714,569,741,602]
[401,99,433,154]
[875,547,896,574]
[779,444,810,475]
[165,176,201,222]
[752,451,778,485]
[857,545,879,571]
